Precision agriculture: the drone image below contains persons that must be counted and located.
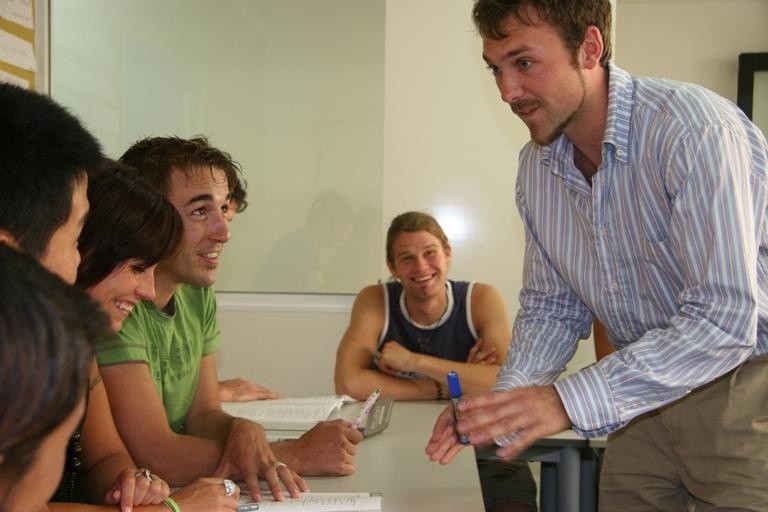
[334,212,512,400]
[1,85,365,511]
[424,0,768,510]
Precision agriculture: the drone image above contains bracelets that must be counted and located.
[432,380,445,400]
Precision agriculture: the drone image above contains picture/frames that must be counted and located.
[737,51,767,141]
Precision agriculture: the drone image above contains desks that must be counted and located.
[531,429,613,511]
[169,392,542,510]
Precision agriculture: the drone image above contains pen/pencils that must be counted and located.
[447,372,470,444]
[237,504,259,512]
[350,388,380,429]
